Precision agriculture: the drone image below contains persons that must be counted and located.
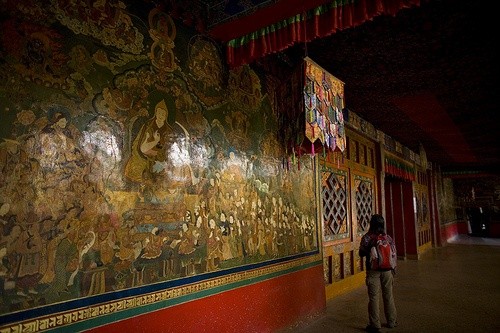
[358,214,400,333]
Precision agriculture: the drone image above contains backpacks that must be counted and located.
[365,232,394,272]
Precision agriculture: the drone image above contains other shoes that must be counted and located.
[389,321,397,326]
[366,325,380,332]
